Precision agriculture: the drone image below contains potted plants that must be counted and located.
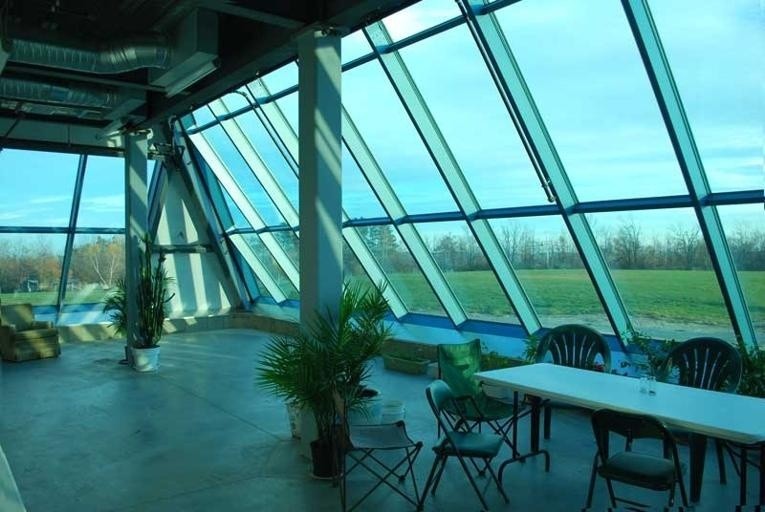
[250,269,397,480]
[102,231,177,373]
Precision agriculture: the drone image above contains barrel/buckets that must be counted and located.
[129,346,161,373]
[286,400,302,439]
[381,399,406,425]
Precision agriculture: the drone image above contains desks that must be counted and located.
[473,362,765,511]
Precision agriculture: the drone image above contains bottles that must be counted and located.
[639,373,647,393]
[648,376,657,394]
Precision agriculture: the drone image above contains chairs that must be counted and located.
[435,338,530,476]
[420,381,509,510]
[0,302,60,363]
[592,408,692,510]
[531,324,612,459]
[625,337,744,501]
[328,380,424,511]
